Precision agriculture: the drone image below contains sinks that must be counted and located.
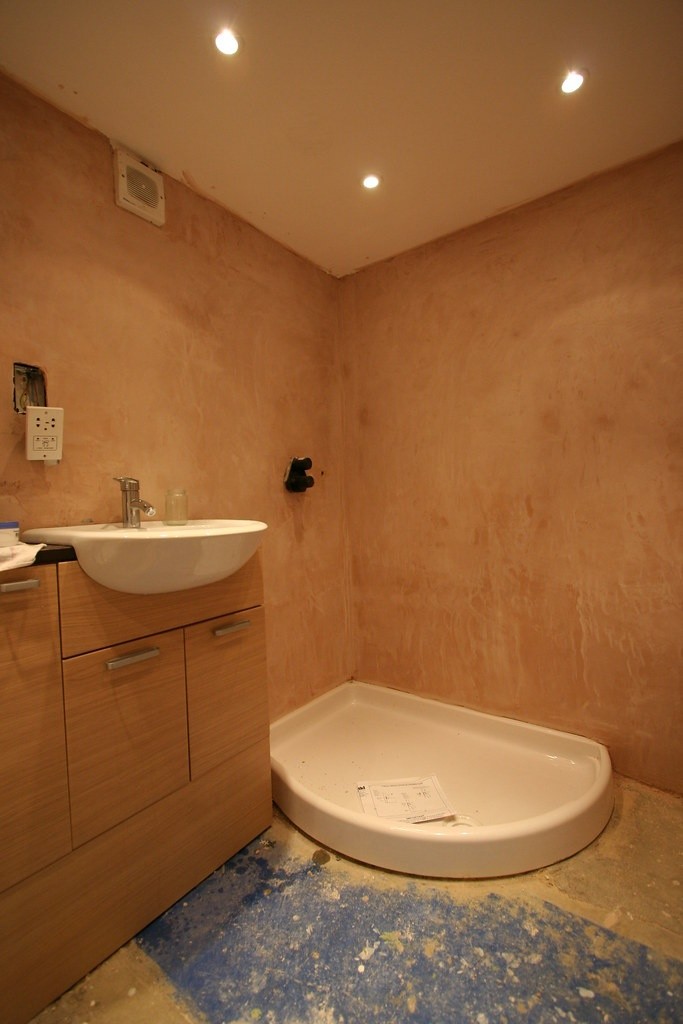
[22,518,269,593]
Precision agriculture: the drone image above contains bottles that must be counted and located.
[165,488,188,525]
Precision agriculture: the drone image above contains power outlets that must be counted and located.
[26,406,64,460]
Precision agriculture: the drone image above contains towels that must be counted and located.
[1,538,47,571]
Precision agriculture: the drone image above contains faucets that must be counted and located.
[113,476,156,528]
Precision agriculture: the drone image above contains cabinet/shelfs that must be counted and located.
[57,551,272,853]
[0,564,72,897]
